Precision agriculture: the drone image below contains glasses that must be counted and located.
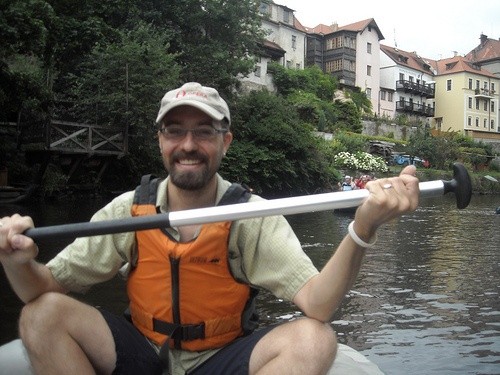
[157,125,226,140]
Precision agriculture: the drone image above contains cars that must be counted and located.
[394,155,425,169]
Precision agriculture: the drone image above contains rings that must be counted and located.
[381,184,392,189]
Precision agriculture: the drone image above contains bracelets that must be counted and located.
[348,220,378,248]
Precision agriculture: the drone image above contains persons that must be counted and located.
[0,83,420,375]
[338,175,356,191]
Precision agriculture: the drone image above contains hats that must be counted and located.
[345,176,350,180]
[156,82,231,127]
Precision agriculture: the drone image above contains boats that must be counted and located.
[333,205,358,219]
